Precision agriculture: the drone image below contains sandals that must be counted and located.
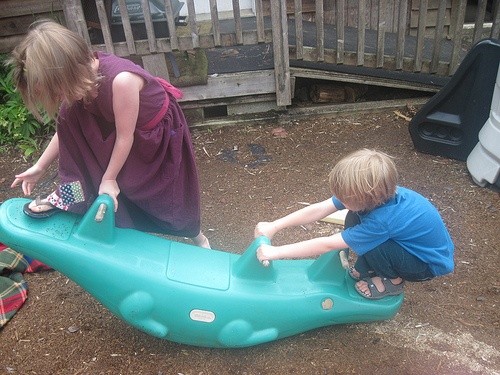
[348,264,406,299]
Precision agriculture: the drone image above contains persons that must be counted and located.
[253,147,454,299]
[6,18,211,251]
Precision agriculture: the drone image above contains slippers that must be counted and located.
[23,193,63,219]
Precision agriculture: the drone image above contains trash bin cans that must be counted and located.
[111,0,184,43]
